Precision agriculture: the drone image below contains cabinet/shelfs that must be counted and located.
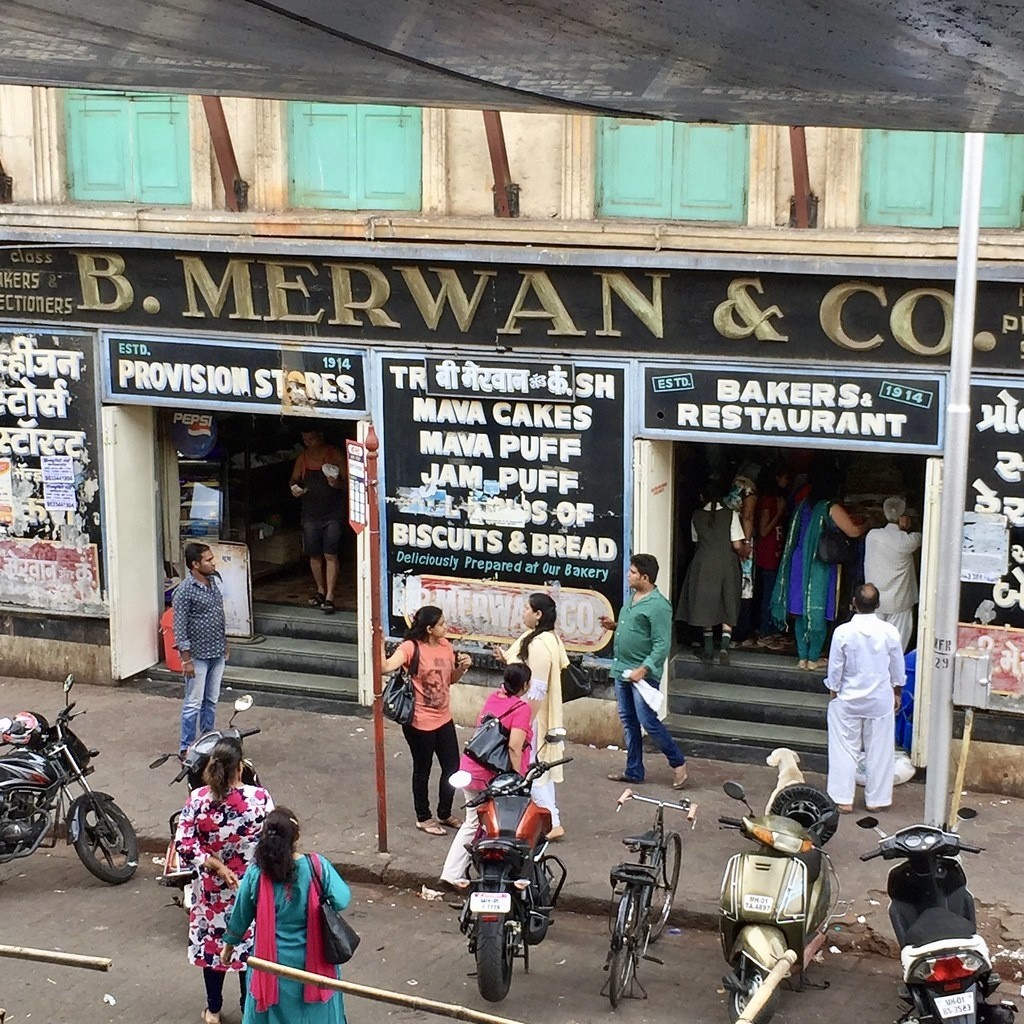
[176,458,231,539]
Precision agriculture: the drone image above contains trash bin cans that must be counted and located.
[160,607,186,672]
[895,647,917,757]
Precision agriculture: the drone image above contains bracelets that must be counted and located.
[183,658,193,664]
[458,667,468,674]
[868,519,872,527]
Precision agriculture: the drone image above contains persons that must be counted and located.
[673,464,877,672]
[171,543,229,755]
[174,738,275,1024]
[823,584,908,814]
[864,495,922,654]
[372,606,471,835]
[492,593,570,841]
[289,421,348,613]
[220,805,351,1024]
[437,662,535,895]
[597,555,687,790]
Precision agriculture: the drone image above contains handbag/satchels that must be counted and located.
[304,853,361,964]
[382,639,419,727]
[818,502,859,565]
[462,700,528,776]
[560,663,593,704]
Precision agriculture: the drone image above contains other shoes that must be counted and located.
[608,772,644,784]
[692,633,828,670]
[437,878,458,894]
[416,819,448,835]
[437,815,465,829]
[200,1007,221,1024]
[673,762,689,789]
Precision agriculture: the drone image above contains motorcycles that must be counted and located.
[148,694,261,915]
[0,673,140,884]
[448,726,574,1002]
[857,808,1018,1024]
[717,781,851,1024]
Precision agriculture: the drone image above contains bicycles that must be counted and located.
[600,789,698,1009]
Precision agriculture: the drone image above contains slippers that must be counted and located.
[835,804,850,814]
[866,805,888,812]
[320,600,335,611]
[308,592,326,607]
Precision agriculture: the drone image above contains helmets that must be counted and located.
[2,711,50,751]
